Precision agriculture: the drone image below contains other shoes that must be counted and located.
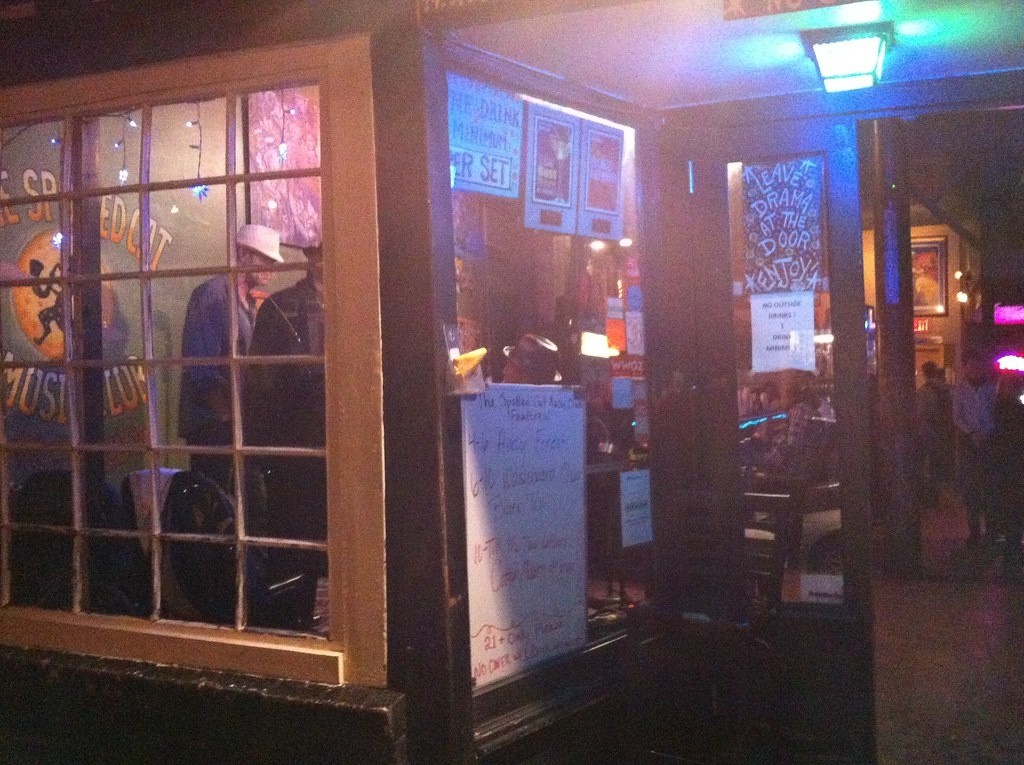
[971,531,1002,552]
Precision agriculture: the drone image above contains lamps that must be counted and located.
[798,19,896,94]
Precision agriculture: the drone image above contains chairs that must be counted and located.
[739,417,836,602]
[12,466,303,629]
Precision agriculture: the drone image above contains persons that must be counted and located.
[494,333,1024,572]
[175,225,344,631]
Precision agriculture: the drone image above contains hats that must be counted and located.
[502,333,563,383]
[237,225,285,263]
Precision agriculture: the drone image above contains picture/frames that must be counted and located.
[911,236,949,318]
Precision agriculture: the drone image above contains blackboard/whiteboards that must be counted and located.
[740,149,828,294]
[460,382,589,689]
